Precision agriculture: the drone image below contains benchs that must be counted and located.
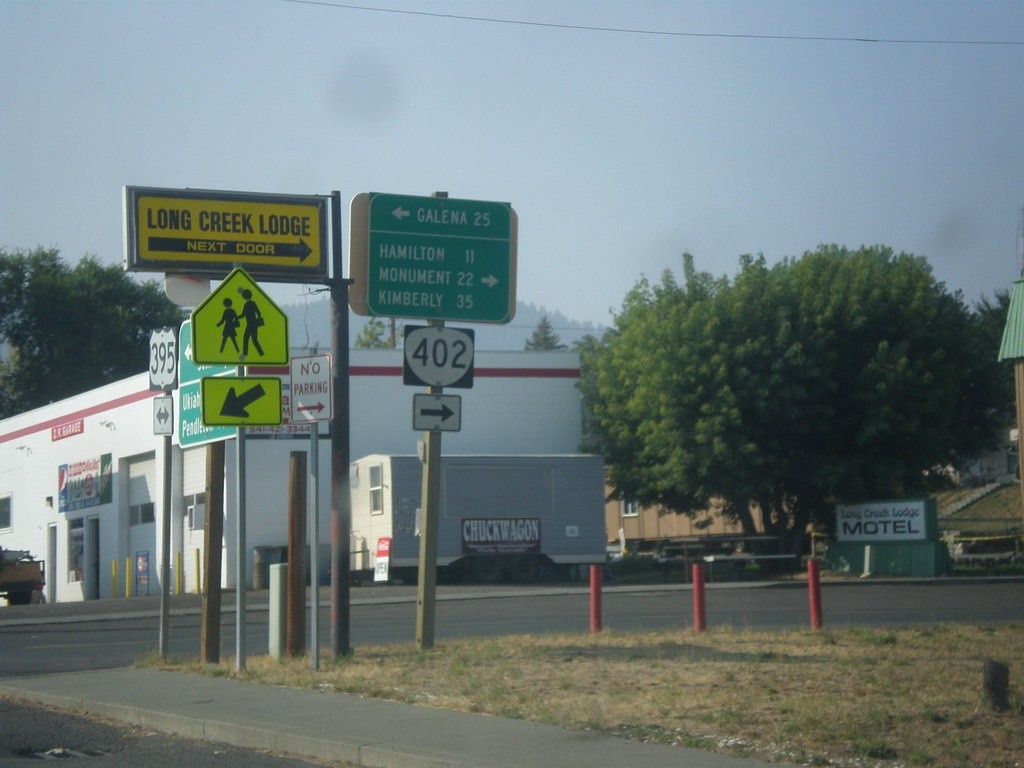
[703,552,796,583]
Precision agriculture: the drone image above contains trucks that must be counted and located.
[0,547,46,605]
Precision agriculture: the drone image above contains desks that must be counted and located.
[671,535,792,584]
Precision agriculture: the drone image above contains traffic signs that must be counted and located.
[123,183,330,273]
[369,192,512,324]
[175,318,245,450]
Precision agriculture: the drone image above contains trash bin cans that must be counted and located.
[252,545,276,589]
